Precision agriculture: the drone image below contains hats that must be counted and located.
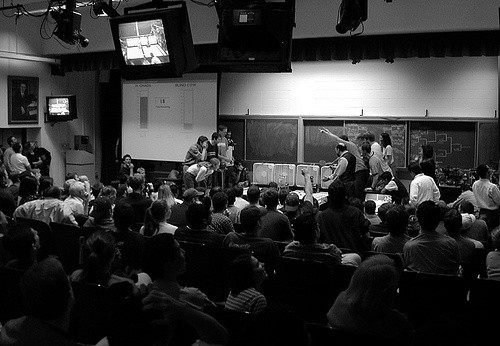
[461,212,475,229]
[284,193,299,211]
[183,188,204,201]
[10,185,19,197]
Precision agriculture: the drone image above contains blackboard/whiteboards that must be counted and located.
[216,115,500,188]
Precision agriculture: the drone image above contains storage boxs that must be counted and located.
[296,165,321,189]
[274,164,297,187]
[253,163,275,185]
[320,165,338,190]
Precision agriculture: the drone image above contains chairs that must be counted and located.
[0,199,500,346]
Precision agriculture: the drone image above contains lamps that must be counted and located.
[92,5,109,18]
[50,10,90,48]
[335,0,369,37]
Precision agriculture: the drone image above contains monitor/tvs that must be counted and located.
[45,94,76,121]
[107,7,185,80]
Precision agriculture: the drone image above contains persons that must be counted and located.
[417,143,435,179]
[13,82,35,119]
[459,185,476,205]
[366,134,382,156]
[182,125,250,189]
[472,164,500,231]
[120,23,167,65]
[378,133,394,174]
[362,143,384,191]
[120,154,134,177]
[356,136,365,147]
[0,167,500,346]
[323,143,356,181]
[0,137,51,183]
[380,171,408,205]
[319,129,369,199]
[408,161,441,204]
